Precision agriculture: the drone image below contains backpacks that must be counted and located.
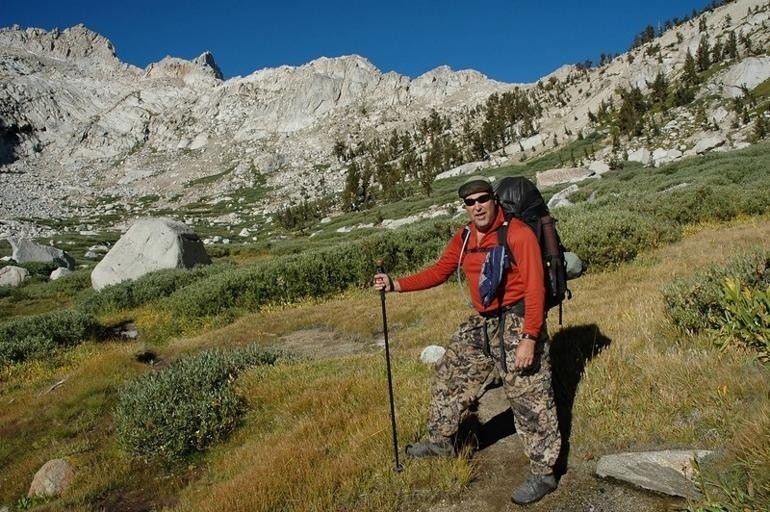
[494,176,566,310]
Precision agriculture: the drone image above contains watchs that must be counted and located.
[522,333,538,342]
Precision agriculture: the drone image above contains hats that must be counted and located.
[459,176,492,196]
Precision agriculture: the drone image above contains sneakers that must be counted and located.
[406,441,455,458]
[511,473,556,504]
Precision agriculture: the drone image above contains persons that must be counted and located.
[375,175,573,505]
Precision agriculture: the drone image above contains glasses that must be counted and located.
[464,195,492,206]
